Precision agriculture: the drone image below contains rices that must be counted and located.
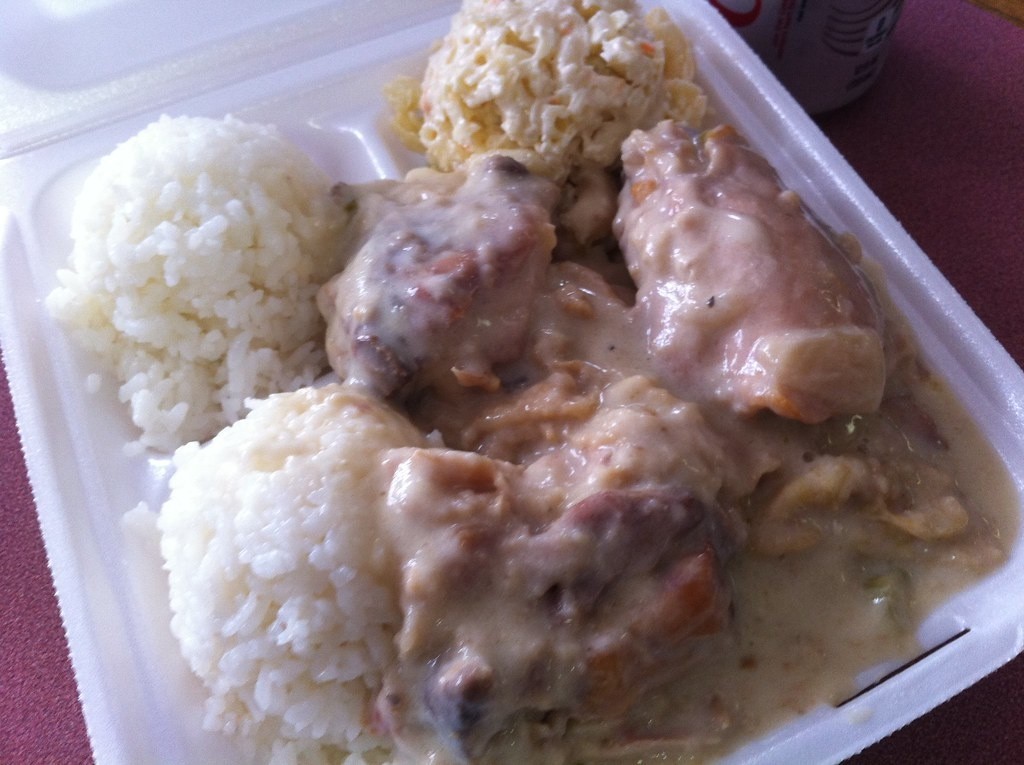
[120,379,466,765]
[46,112,351,452]
